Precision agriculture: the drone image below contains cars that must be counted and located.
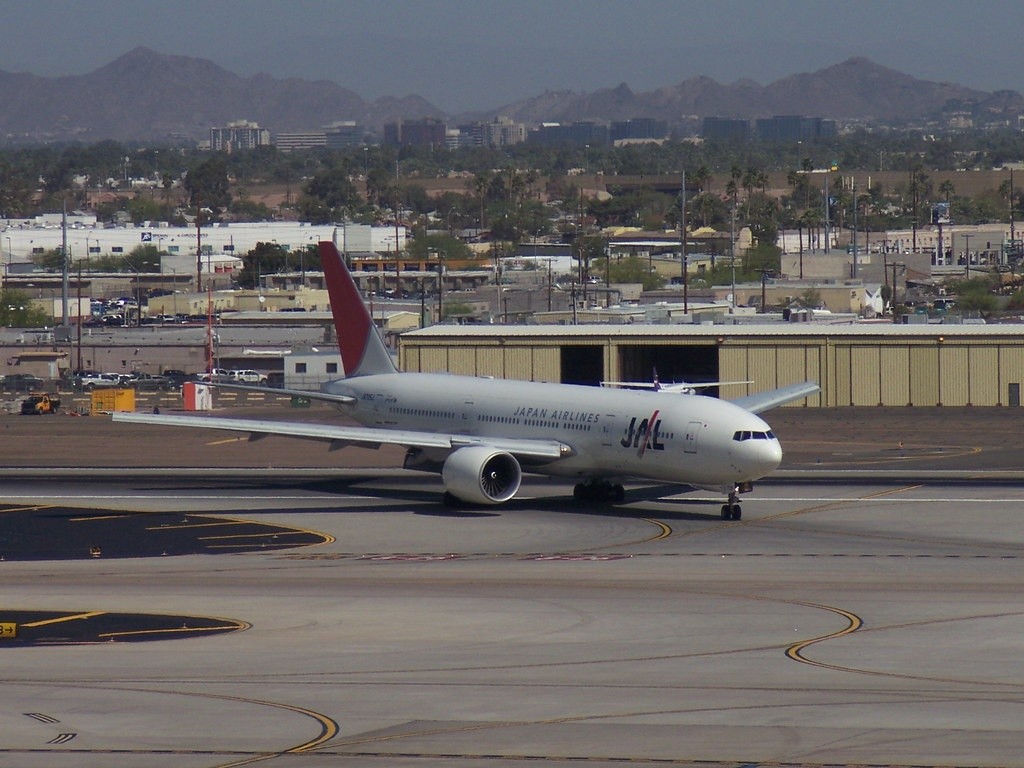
[73,368,268,391]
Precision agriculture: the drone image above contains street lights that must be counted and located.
[7,237,11,273]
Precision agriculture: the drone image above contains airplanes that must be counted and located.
[93,241,822,522]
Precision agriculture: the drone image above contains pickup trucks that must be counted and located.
[21,396,61,414]
